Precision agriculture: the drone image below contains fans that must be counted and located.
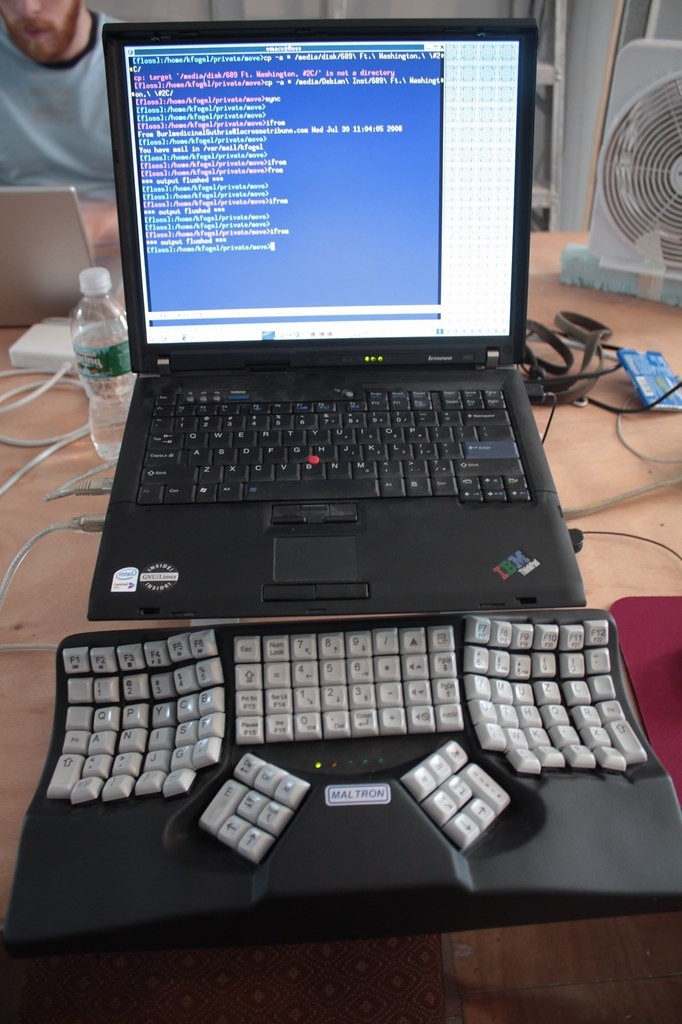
[588,37,682,282]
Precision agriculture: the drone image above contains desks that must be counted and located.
[0,228,682,1024]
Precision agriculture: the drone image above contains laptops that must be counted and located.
[86,17,587,622]
[0,185,124,329]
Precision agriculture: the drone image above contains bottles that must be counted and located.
[72,267,135,463]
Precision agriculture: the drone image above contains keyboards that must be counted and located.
[7,610,681,959]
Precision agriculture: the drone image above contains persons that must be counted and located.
[0,0,128,203]
